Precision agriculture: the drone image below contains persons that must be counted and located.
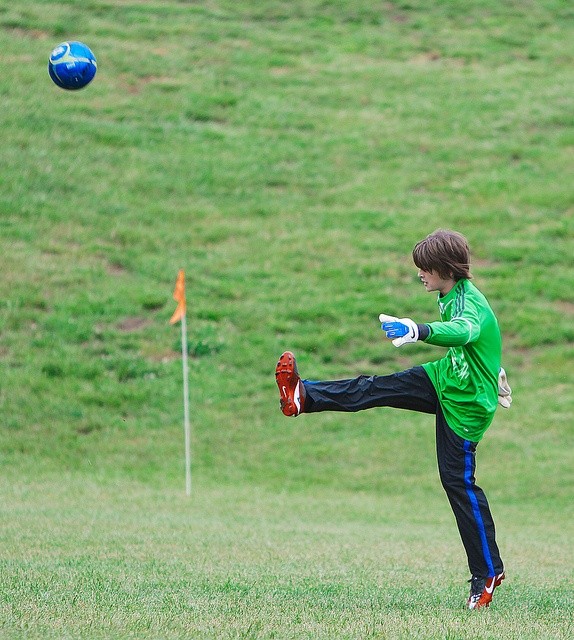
[276,230,512,609]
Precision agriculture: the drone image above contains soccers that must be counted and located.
[47,41,97,91]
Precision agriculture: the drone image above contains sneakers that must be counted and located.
[276,351,305,416]
[463,571,505,610]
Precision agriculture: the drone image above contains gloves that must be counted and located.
[497,367,513,409]
[379,313,429,348]
